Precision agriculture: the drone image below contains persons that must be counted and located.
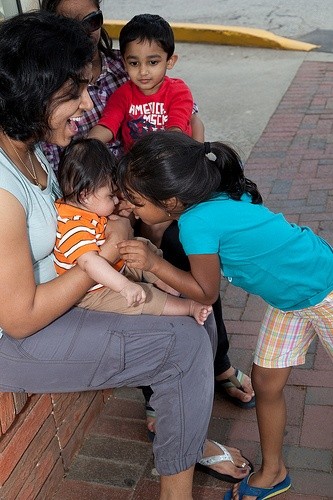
[0,0,254,499]
[119,129,333,500]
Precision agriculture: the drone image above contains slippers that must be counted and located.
[223,472,291,500]
[218,368,256,409]
[145,401,156,442]
[196,439,255,483]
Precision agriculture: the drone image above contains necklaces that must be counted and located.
[4,132,42,189]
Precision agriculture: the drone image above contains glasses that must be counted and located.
[81,10,103,33]
